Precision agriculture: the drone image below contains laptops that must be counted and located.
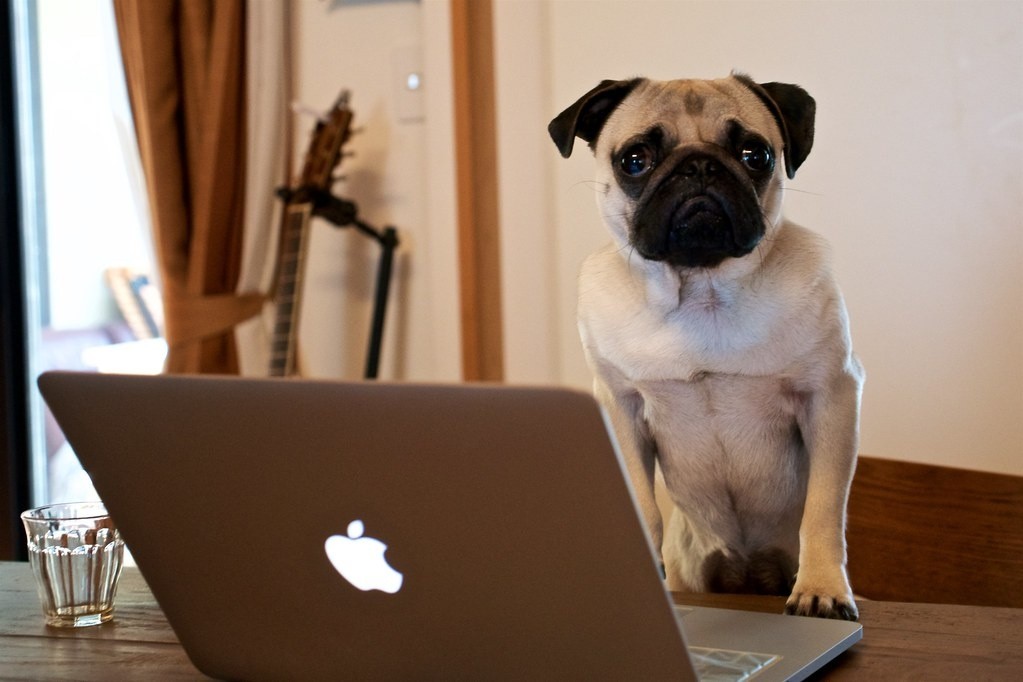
[37,370,864,682]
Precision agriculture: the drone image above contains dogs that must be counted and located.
[548,70,866,622]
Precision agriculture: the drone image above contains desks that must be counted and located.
[0,559,1023,682]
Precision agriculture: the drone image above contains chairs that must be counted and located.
[845,452,1022,607]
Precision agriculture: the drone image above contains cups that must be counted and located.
[21,501,125,627]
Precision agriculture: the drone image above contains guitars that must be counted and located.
[264,83,364,378]
